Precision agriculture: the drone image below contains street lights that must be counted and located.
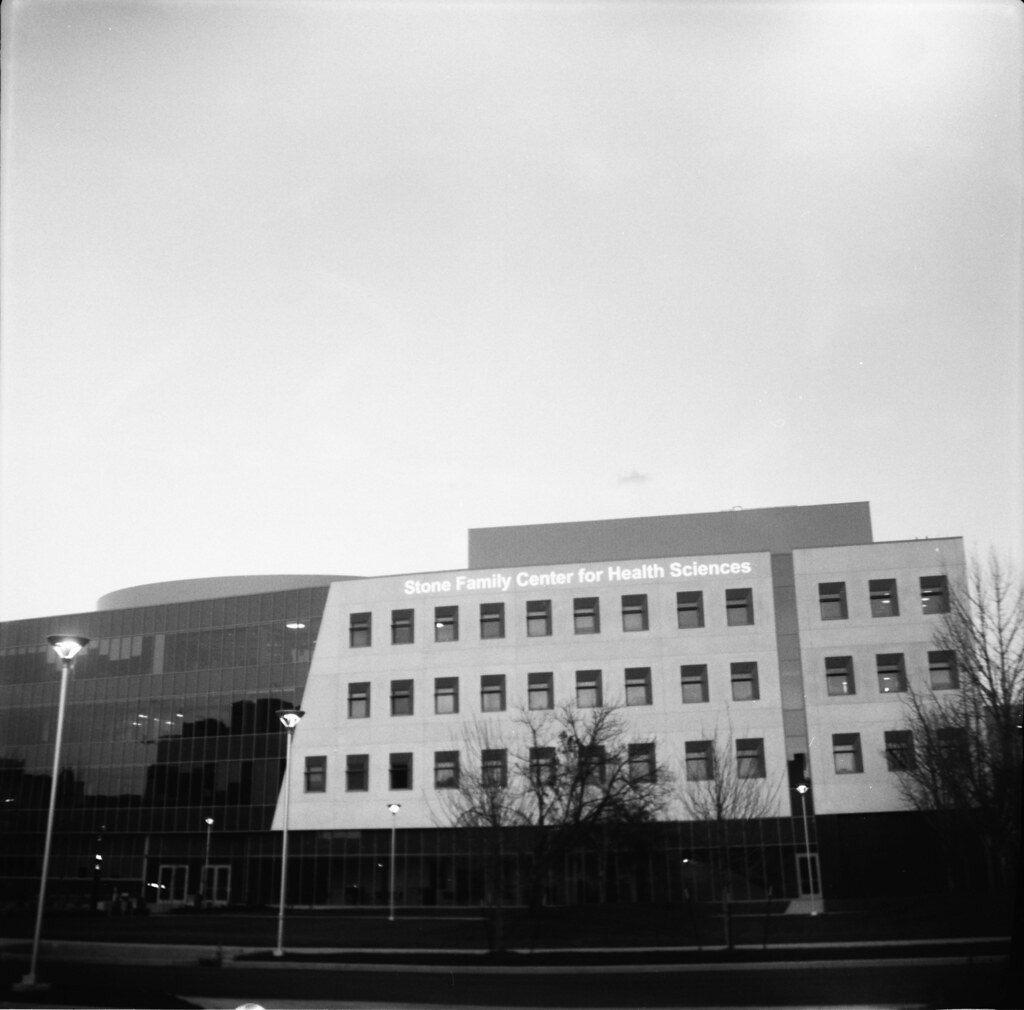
[17,633,90,995]
[792,785,821,917]
[269,708,306,959]
[197,816,216,916]
[383,804,401,921]
